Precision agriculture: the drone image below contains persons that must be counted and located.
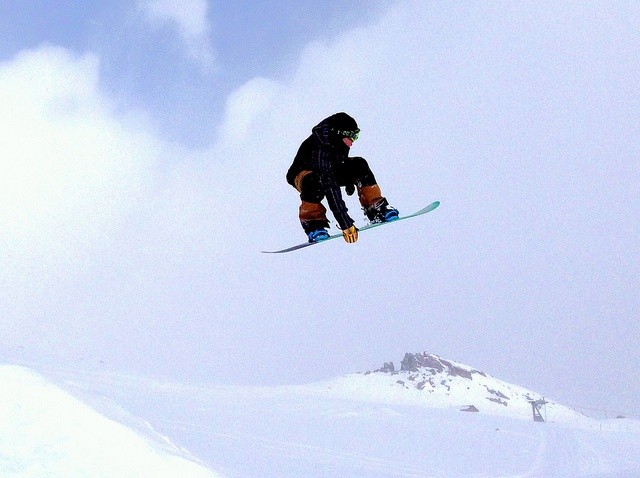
[286,112,400,242]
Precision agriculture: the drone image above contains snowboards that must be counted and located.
[261,200,440,254]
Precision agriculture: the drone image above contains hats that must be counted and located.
[328,112,358,133]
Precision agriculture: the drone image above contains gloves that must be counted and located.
[342,226,360,243]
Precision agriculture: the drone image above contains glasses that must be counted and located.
[342,128,360,141]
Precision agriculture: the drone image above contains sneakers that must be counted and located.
[368,205,399,221]
[309,229,329,241]
